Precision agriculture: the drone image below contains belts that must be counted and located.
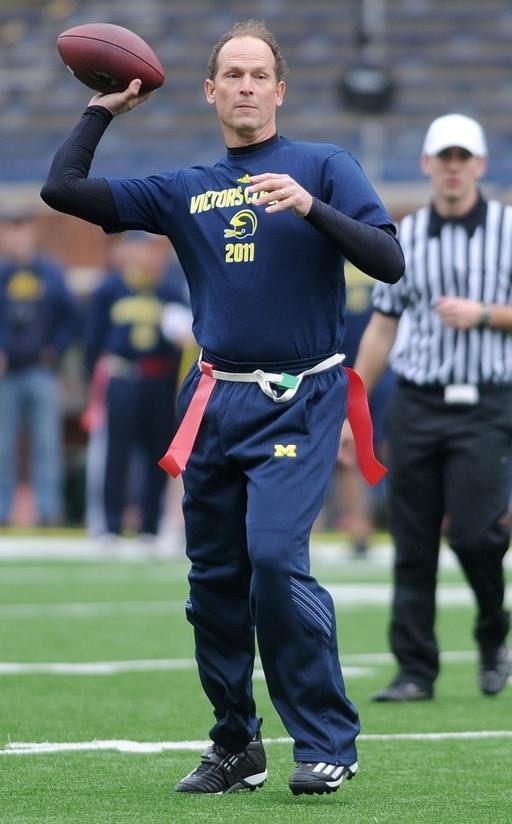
[394,373,512,406]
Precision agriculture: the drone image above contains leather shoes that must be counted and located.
[477,639,510,696]
[371,676,434,702]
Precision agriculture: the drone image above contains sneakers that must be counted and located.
[285,756,361,796]
[172,714,273,795]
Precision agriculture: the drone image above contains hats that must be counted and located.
[419,111,491,161]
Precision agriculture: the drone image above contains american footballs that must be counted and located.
[57,22,164,94]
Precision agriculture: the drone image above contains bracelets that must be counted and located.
[477,302,491,327]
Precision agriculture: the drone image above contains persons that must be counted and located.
[39,21,408,800]
[333,110,512,701]
